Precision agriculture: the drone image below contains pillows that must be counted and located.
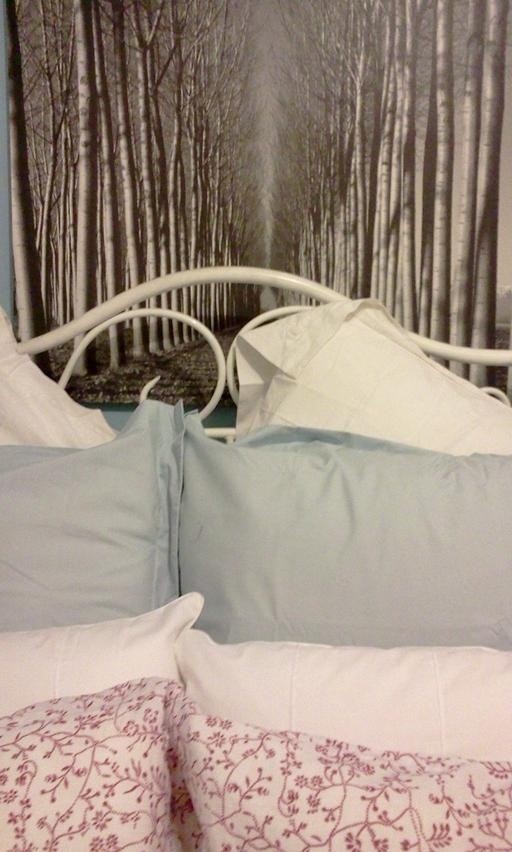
[0,300,512,852]
